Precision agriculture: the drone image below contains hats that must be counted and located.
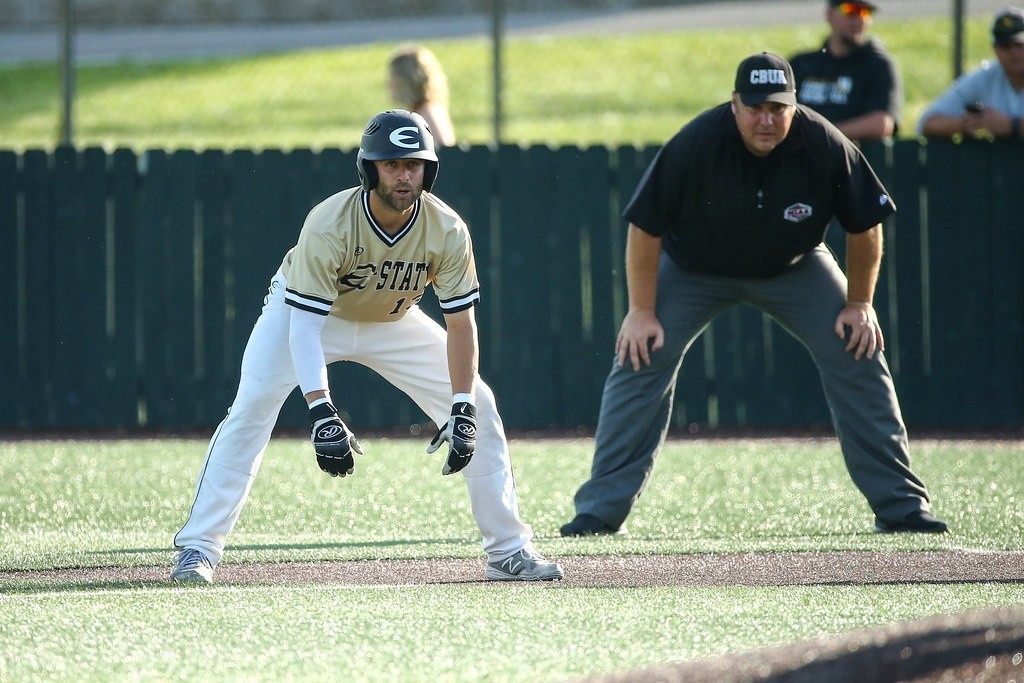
[829,0,877,13]
[735,52,796,105]
[991,12,1024,46]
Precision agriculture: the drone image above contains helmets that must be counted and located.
[356,109,440,193]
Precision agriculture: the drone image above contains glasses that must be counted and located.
[831,2,873,24]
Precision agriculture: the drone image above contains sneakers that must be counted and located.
[170,549,213,583]
[875,511,946,533]
[560,522,612,537]
[484,545,564,579]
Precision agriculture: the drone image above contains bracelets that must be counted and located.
[1010,116,1020,135]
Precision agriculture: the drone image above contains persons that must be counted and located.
[560,52,946,537]
[388,48,456,146]
[784,0,902,137]
[169,110,564,584]
[918,10,1024,136]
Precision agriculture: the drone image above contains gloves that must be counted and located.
[427,402,478,475]
[308,402,363,478]
